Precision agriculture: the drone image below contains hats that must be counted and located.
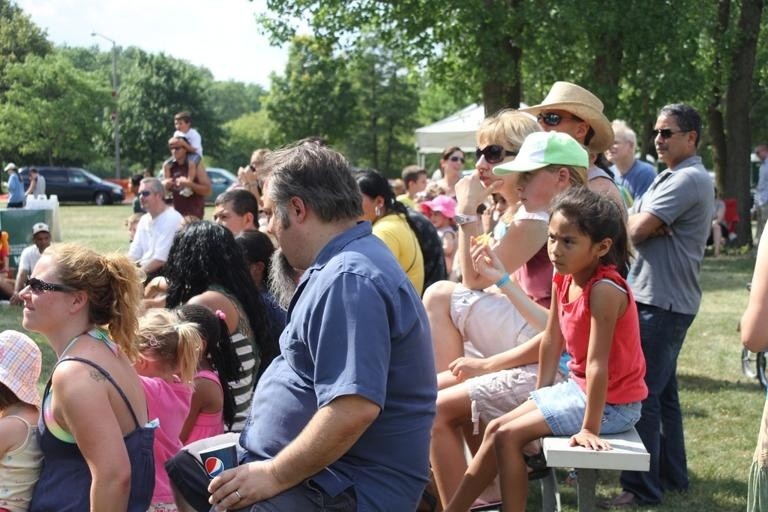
[31,222,49,236]
[419,194,457,225]
[491,131,590,177]
[3,162,17,172]
[517,80,615,154]
[0,329,46,407]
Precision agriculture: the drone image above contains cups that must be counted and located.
[26,192,58,210]
[197,442,238,483]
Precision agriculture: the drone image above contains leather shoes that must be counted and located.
[596,490,639,509]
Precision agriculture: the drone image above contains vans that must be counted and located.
[16,165,127,207]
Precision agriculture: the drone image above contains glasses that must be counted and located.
[139,191,150,196]
[651,128,681,139]
[536,112,574,126]
[24,276,74,294]
[475,143,516,165]
[448,156,465,164]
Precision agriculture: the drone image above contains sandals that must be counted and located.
[523,449,550,480]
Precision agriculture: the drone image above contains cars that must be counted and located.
[202,165,240,203]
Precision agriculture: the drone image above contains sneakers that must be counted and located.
[179,187,193,197]
[165,190,174,200]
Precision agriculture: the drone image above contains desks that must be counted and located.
[0,203,61,279]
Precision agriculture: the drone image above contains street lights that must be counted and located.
[91,31,121,180]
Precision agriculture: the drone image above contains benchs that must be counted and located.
[536,427,652,511]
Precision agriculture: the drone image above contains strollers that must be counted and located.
[739,283,768,394]
